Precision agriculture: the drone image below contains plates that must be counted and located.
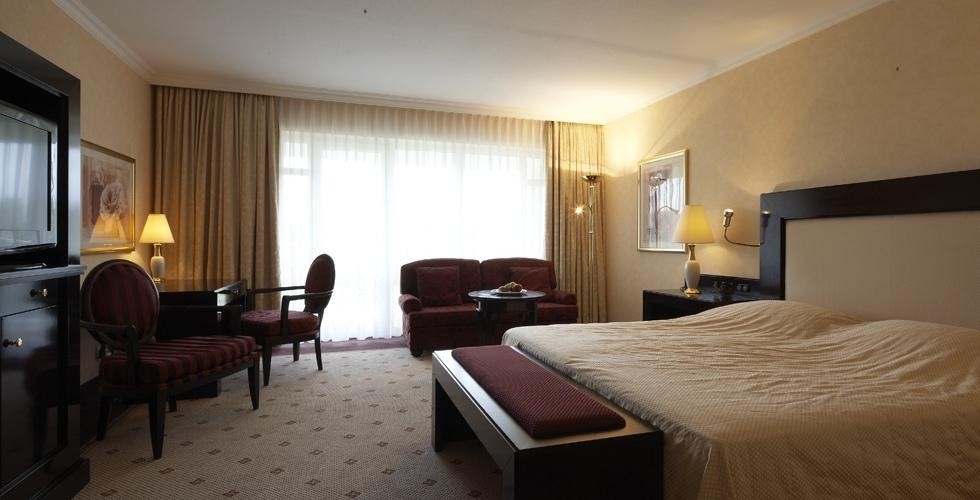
[494,289,528,295]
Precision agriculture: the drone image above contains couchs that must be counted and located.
[398,255,579,359]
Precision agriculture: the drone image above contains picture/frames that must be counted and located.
[636,149,689,254]
[81,139,139,258]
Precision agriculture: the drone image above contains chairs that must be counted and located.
[82,260,259,460]
[240,253,336,385]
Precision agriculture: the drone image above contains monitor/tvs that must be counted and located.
[0,100,58,272]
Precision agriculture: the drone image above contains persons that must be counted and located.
[83,155,130,247]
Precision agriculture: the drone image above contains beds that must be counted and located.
[500,171,980,498]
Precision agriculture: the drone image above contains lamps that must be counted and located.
[136,211,173,281]
[673,207,718,297]
[722,207,759,249]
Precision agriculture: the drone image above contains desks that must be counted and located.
[153,279,246,399]
[466,289,546,341]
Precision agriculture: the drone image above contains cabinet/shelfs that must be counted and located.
[0,259,92,499]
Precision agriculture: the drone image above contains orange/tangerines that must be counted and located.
[499,282,523,292]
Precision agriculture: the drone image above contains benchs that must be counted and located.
[428,345,664,498]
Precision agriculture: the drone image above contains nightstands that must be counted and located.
[641,273,756,320]
